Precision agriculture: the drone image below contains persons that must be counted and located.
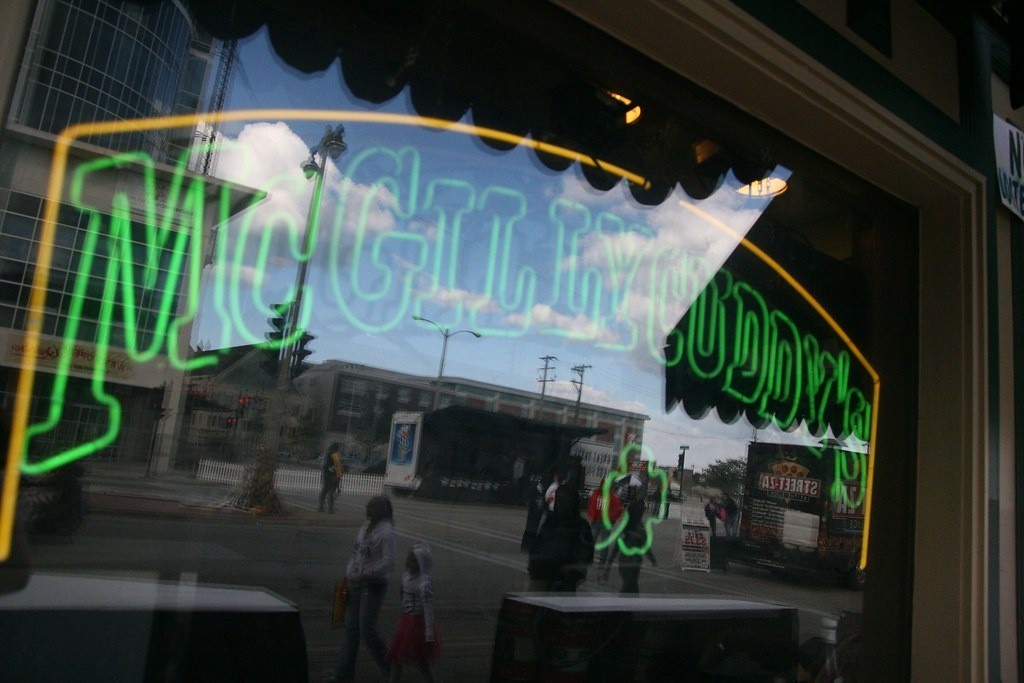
[384,544,445,682]
[334,495,395,682]
[520,466,658,598]
[705,497,720,536]
[317,443,345,514]
[720,492,738,536]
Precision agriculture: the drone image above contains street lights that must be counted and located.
[412,314,483,415]
[237,120,347,517]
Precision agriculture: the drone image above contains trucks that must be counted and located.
[741,439,866,590]
